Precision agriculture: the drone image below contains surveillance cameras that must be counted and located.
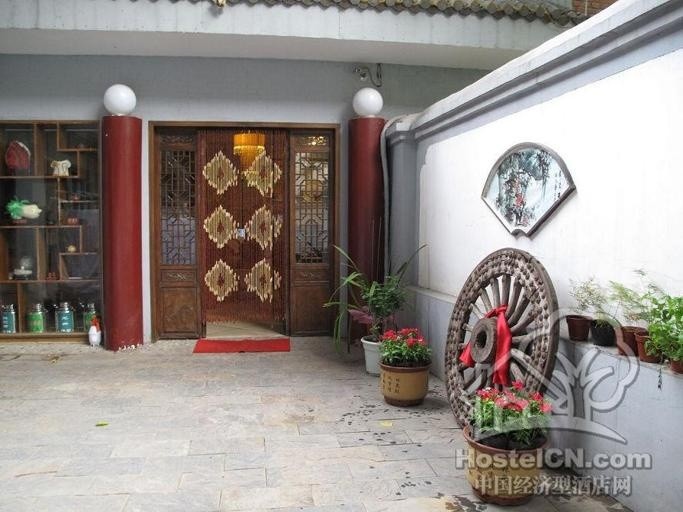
[360,70,369,81]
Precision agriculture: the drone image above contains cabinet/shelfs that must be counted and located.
[0,117,105,345]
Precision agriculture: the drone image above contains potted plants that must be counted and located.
[563,266,682,373]
[320,238,428,377]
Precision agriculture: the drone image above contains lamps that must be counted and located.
[102,82,137,117]
[350,88,384,119]
[231,128,265,158]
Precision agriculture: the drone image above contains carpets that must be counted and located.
[192,336,292,354]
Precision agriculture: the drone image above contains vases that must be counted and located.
[377,361,430,408]
[459,423,542,506]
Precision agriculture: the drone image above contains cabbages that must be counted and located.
[6,196,42,221]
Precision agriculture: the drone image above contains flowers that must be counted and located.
[460,376,552,449]
[378,326,433,366]
